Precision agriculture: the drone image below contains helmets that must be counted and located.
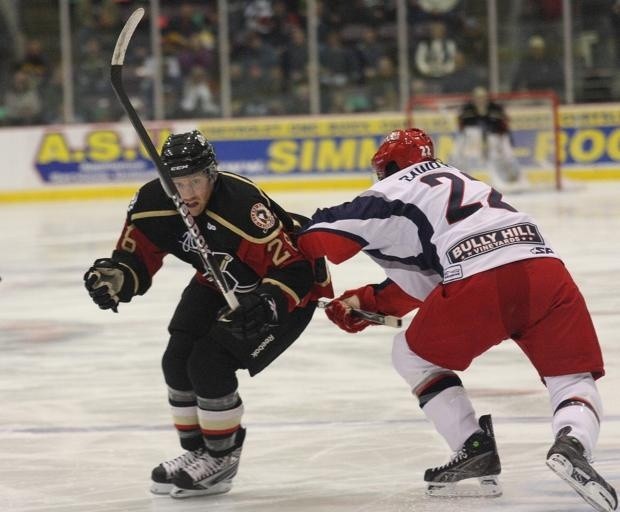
[156,129,217,184]
[372,124,433,177]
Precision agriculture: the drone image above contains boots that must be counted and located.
[422,413,501,486]
[150,423,247,491]
[544,427,617,511]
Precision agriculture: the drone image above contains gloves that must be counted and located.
[218,292,279,339]
[82,256,128,316]
[323,283,377,334]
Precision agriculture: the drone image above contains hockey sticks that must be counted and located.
[109,7,240,312]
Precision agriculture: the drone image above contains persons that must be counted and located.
[81,130,334,491]
[448,86,525,191]
[284,125,619,510]
